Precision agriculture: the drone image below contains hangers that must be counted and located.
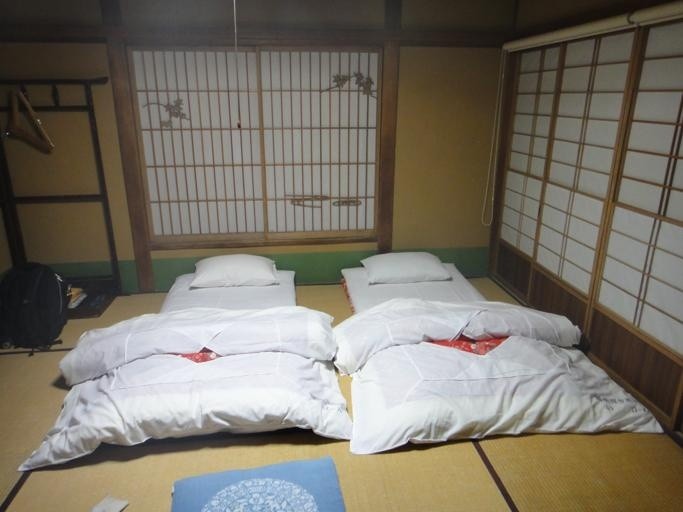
[0,87,54,154]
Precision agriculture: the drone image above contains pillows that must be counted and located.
[191,254,279,289]
[359,251,453,285]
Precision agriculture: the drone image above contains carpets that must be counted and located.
[172,457,345,512]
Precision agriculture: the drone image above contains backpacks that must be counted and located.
[0,262,64,356]
